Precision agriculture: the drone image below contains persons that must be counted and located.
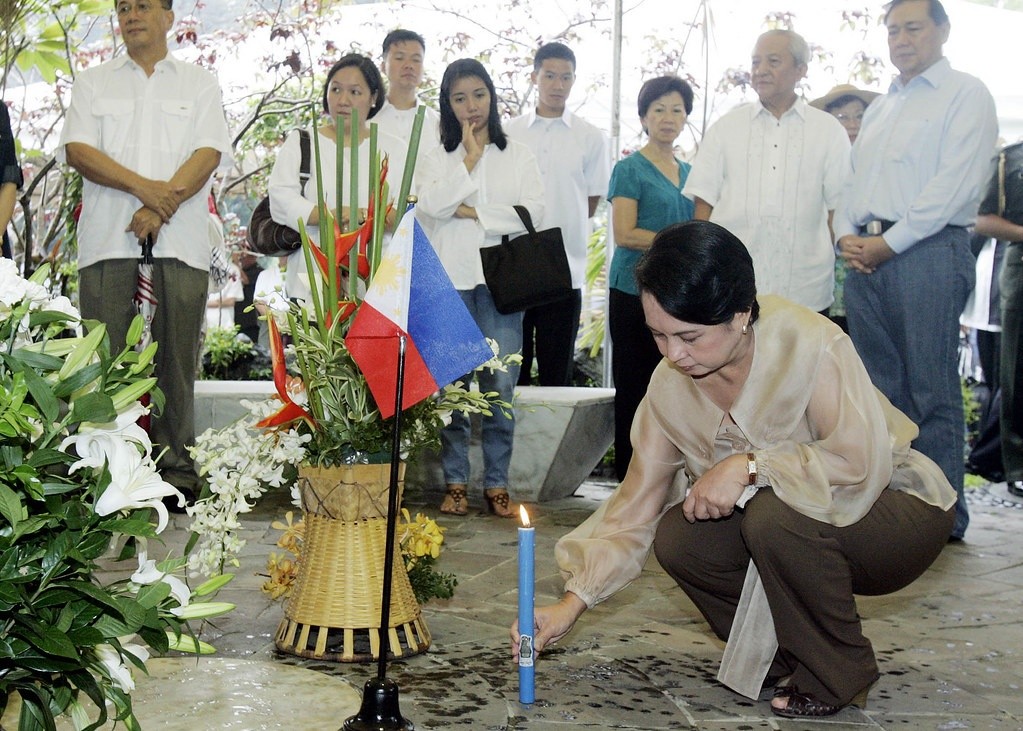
[269,54,417,433]
[959,142,1023,496]
[606,76,695,481]
[808,84,880,332]
[365,29,441,157]
[834,0,998,540]
[681,29,852,321]
[57,0,233,512]
[416,59,546,518]
[0,100,24,260]
[199,190,292,381]
[511,219,956,717]
[500,43,606,387]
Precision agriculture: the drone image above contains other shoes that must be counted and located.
[162,485,199,515]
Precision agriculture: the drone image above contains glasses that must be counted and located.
[836,113,863,123]
[116,2,170,17]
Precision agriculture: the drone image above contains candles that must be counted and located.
[516,503,536,704]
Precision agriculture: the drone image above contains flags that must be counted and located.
[345,206,496,419]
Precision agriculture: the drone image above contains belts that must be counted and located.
[858,218,897,236]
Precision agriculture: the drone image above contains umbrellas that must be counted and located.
[133,233,158,430]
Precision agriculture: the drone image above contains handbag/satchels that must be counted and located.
[480,206,574,315]
[247,127,311,257]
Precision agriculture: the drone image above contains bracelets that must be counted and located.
[747,452,757,485]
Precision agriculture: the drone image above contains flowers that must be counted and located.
[0,253,234,731]
[185,106,522,602]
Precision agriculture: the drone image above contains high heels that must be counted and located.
[483,487,519,519]
[770,674,881,718]
[440,488,469,515]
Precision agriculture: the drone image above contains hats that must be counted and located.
[808,84,885,112]
